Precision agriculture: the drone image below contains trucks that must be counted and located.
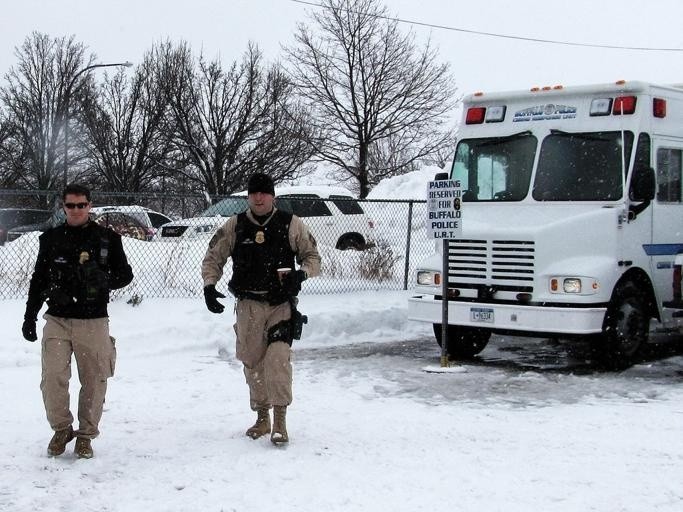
[403,80,683,376]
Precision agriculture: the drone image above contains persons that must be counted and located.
[20,183,135,459]
[196,171,322,444]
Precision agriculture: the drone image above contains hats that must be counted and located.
[246,173,277,197]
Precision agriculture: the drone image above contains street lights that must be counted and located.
[58,61,134,207]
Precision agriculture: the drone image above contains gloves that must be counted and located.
[90,268,111,291]
[20,317,39,343]
[279,269,308,294]
[201,283,226,316]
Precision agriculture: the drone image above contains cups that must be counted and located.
[82,259,99,301]
[276,267,292,285]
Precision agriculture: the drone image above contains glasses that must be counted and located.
[63,201,88,209]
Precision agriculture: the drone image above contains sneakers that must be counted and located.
[45,425,74,457]
[73,435,93,460]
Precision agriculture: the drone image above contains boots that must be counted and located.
[269,403,290,446]
[243,407,271,441]
